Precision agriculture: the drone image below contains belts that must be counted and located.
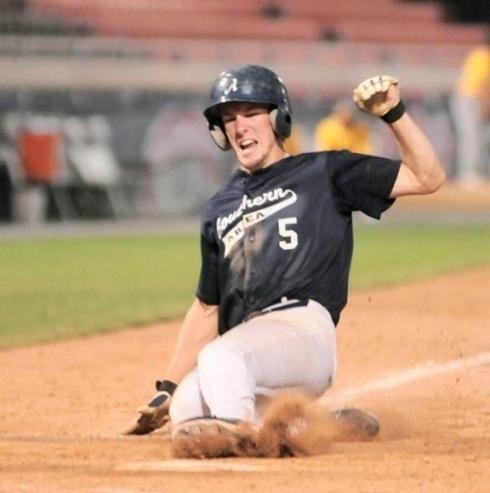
[244,301,308,318]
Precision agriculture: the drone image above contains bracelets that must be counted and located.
[154,377,176,395]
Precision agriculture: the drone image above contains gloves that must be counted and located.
[353,76,404,124]
[127,381,175,434]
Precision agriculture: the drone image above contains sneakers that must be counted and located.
[287,406,378,446]
[171,416,257,446]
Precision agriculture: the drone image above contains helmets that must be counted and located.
[203,65,291,150]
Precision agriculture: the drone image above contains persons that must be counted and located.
[1,0,460,221]
[450,23,490,187]
[116,62,447,442]
